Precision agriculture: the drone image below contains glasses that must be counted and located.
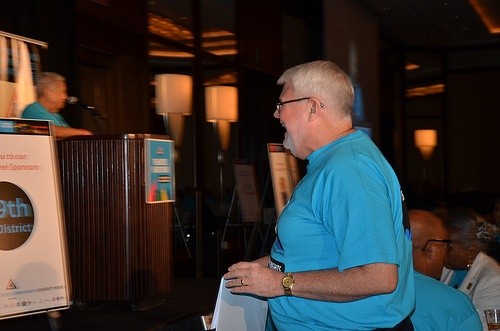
[277,97,325,113]
[422,239,452,252]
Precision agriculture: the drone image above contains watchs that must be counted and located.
[282,272,294,297]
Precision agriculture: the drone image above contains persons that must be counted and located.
[21,72,92,137]
[224,61,415,331]
[407,206,500,331]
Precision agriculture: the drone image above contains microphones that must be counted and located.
[68,96,101,115]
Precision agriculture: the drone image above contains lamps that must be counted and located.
[155,74,193,115]
[204,86,238,123]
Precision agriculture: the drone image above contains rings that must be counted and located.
[240,278,245,285]
